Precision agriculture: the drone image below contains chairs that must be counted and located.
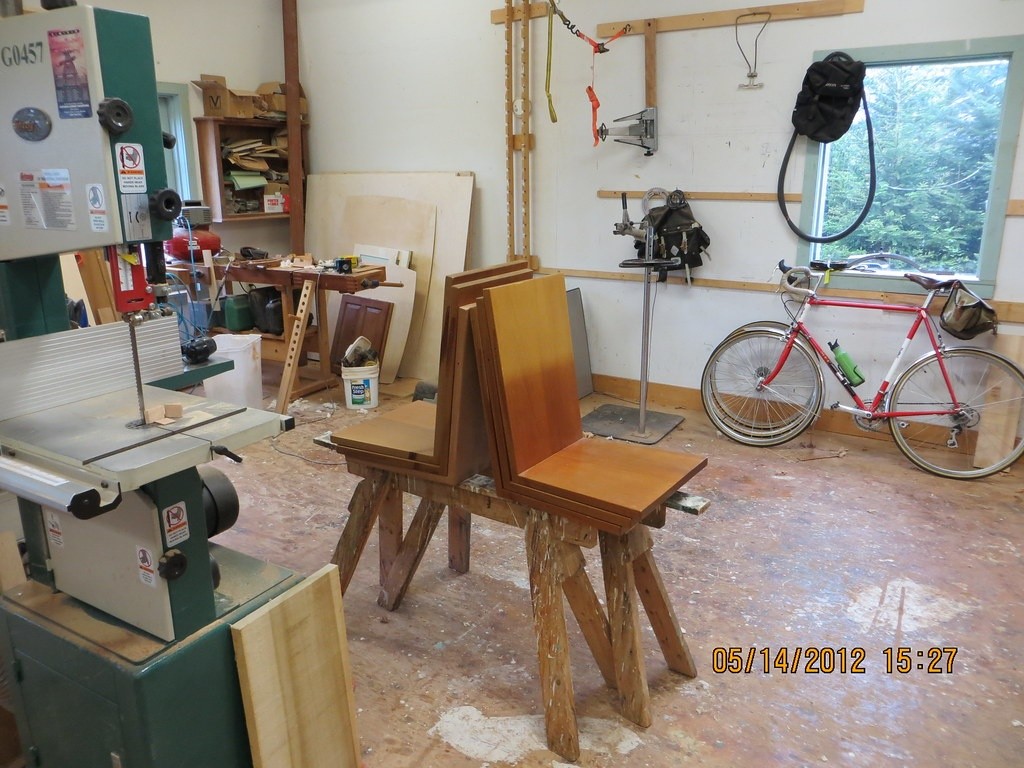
[330,260,710,536]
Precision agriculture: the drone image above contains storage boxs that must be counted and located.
[255,81,307,114]
[190,74,260,119]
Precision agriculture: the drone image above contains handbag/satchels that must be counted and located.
[792,59,866,143]
[635,202,710,271]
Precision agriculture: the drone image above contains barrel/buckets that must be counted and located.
[340,357,380,409]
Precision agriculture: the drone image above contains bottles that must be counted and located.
[828,338,865,386]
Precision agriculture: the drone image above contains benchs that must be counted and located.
[330,455,699,763]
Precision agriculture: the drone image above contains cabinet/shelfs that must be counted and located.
[193,117,311,223]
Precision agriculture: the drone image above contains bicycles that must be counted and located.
[701,251,1023,479]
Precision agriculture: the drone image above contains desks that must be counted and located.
[166,254,386,415]
